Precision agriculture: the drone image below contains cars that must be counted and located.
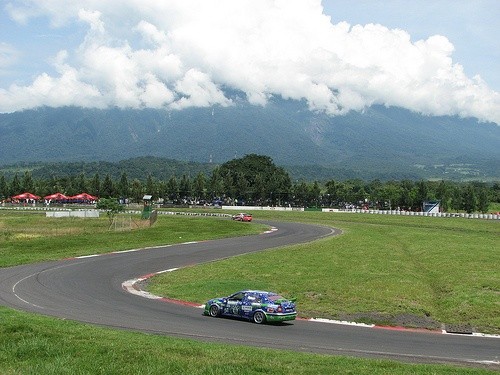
[232,212,252,223]
[204,289,298,324]
